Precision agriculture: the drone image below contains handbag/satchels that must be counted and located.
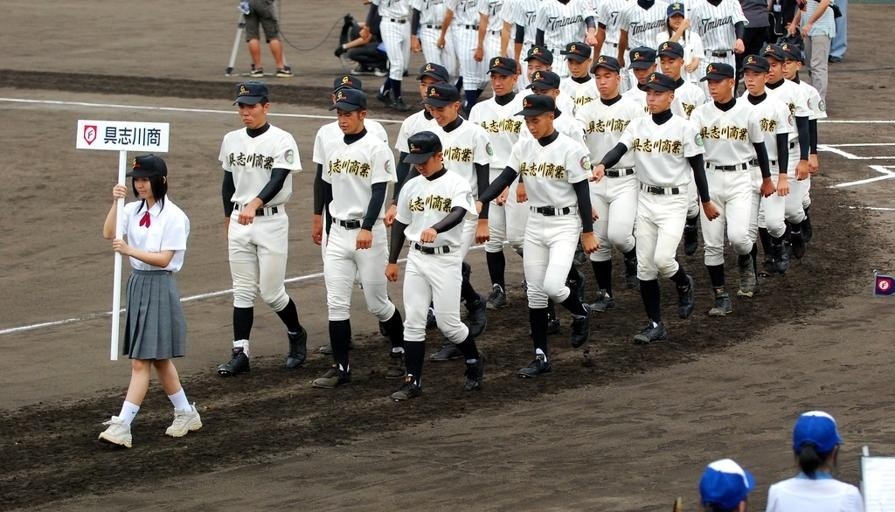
[833,5,841,18]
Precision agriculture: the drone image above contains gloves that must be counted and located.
[335,45,347,57]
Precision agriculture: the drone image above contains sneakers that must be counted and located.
[464,294,487,338]
[285,329,306,369]
[829,55,840,63]
[166,404,202,437]
[709,287,732,316]
[633,319,668,345]
[737,257,757,298]
[575,270,585,303]
[250,65,264,77]
[625,256,639,288]
[98,416,133,449]
[430,340,464,361]
[791,233,805,258]
[548,319,561,334]
[393,97,412,111]
[376,65,388,76]
[486,287,508,309]
[350,63,375,75]
[276,65,293,77]
[392,382,421,401]
[217,347,249,375]
[677,275,694,319]
[758,255,774,278]
[772,241,790,275]
[683,225,698,256]
[589,289,615,312]
[571,304,590,348]
[464,354,485,392]
[800,220,812,242]
[516,353,553,378]
[313,366,352,389]
[376,87,392,105]
[385,349,407,379]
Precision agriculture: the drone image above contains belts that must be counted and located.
[492,30,500,36]
[706,162,747,172]
[332,216,360,229]
[234,204,278,216]
[750,159,776,167]
[551,49,566,54]
[648,186,679,195]
[391,19,406,23]
[415,243,450,254]
[613,43,617,47]
[705,51,733,58]
[530,206,570,217]
[427,24,441,29]
[603,169,633,177]
[789,142,794,149]
[466,25,477,30]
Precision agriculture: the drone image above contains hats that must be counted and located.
[486,56,516,75]
[524,46,553,65]
[782,44,801,61]
[125,154,166,178]
[232,80,268,105]
[640,72,675,91]
[419,82,458,107]
[667,2,685,17]
[699,459,755,507]
[590,55,620,73]
[329,89,368,112]
[514,94,555,117]
[416,63,449,82]
[403,131,441,164]
[333,75,362,94]
[759,44,784,61]
[564,42,591,62]
[655,41,684,58]
[794,410,844,455]
[628,46,656,69]
[700,63,734,82]
[525,71,560,89]
[742,54,770,72]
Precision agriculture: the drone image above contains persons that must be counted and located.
[239,0,295,77]
[763,408,866,512]
[310,1,848,400]
[698,456,758,511]
[97,154,204,450]
[214,82,308,379]
